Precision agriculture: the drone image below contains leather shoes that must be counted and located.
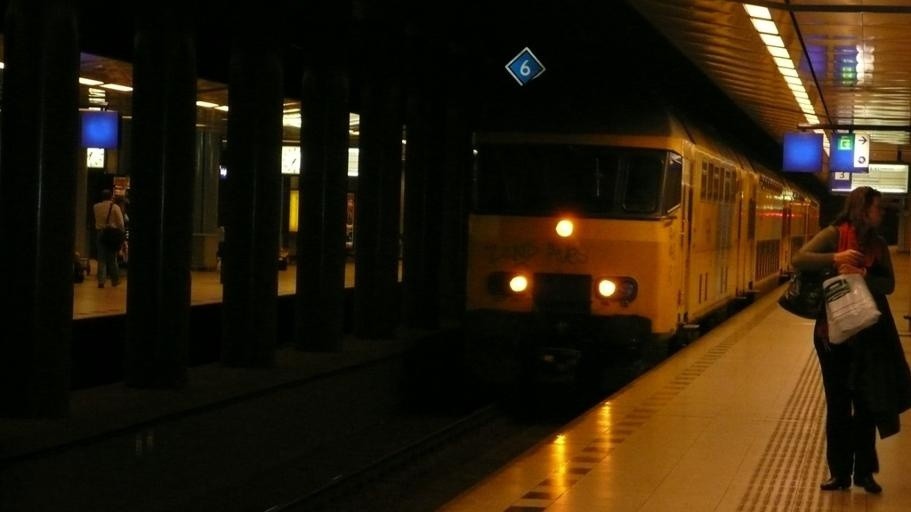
[853,471,883,495]
[819,474,852,491]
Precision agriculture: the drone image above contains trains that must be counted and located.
[465,95,821,360]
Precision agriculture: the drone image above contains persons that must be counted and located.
[791,186,911,493]
[93,185,129,288]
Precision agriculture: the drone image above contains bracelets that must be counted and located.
[862,267,867,276]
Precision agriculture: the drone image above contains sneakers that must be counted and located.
[98,280,121,289]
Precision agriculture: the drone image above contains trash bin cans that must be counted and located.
[193,233,217,272]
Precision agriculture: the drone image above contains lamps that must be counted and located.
[780,120,910,175]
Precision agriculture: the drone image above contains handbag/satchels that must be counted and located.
[777,265,829,322]
[821,272,883,347]
[98,225,125,252]
